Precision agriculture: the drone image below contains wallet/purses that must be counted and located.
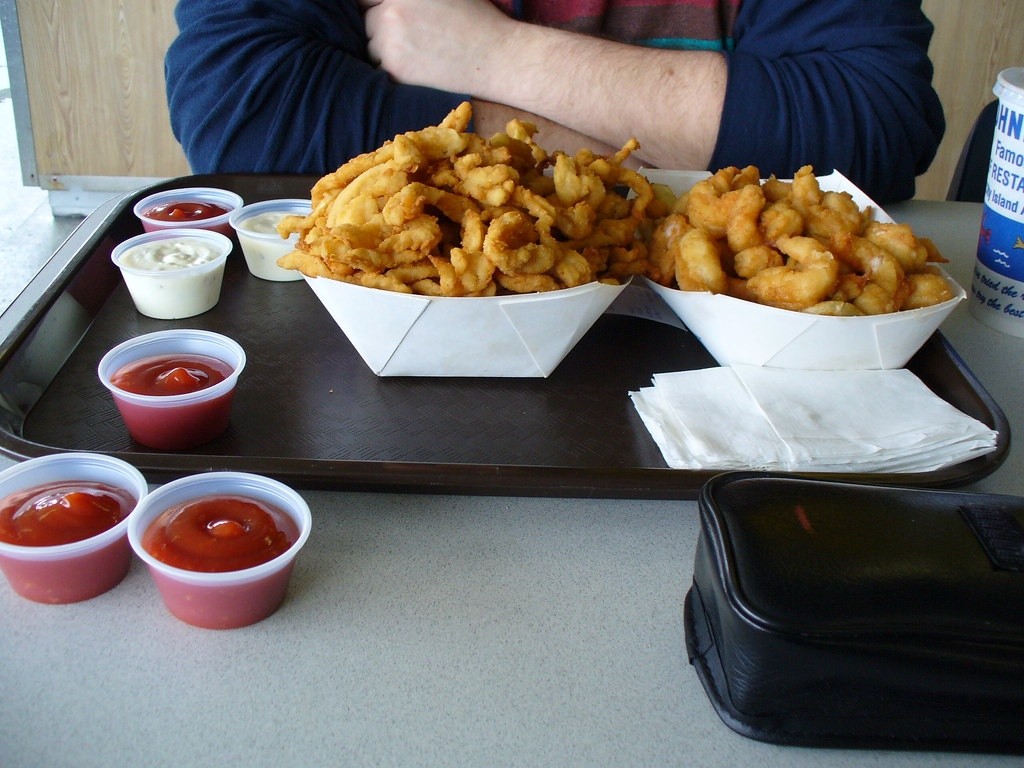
[681,470,1024,757]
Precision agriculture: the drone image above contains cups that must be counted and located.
[968,66,1024,339]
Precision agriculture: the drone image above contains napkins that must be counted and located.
[626,367,999,475]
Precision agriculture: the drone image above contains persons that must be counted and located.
[163,0,948,206]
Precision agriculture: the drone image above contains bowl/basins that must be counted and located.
[133,187,244,240]
[229,199,313,281]
[0,453,148,604]
[98,329,246,450]
[110,228,233,320]
[128,472,312,629]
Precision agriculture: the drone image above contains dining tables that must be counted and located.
[1,196,1024,767]
